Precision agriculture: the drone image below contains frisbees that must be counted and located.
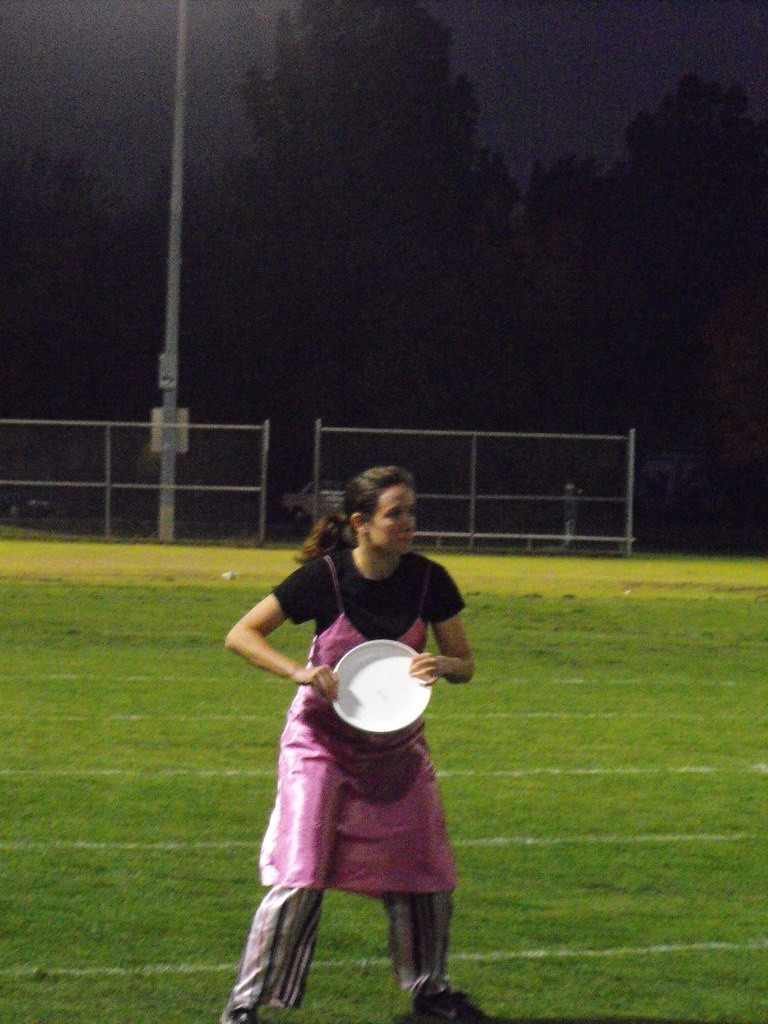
[332,639,433,734]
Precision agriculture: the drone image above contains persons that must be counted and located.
[221,467,494,1024]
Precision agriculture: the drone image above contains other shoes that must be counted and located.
[413,986,493,1024]
[220,1007,260,1024]
